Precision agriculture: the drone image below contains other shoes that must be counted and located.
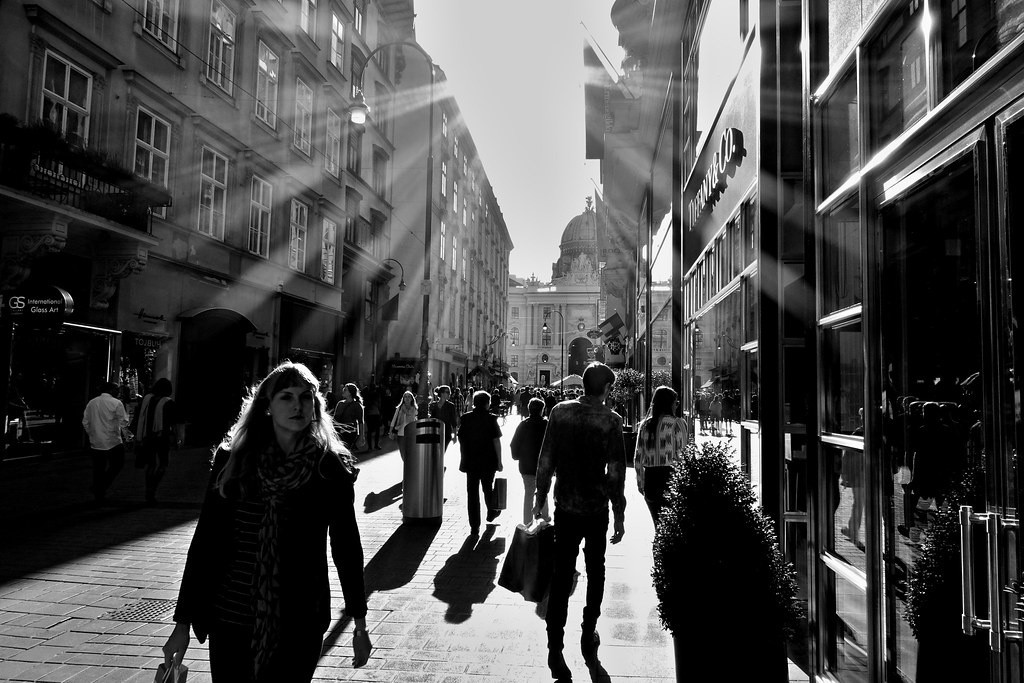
[487,509,501,522]
[548,648,564,667]
[471,528,479,536]
[582,632,600,648]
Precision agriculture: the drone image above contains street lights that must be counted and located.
[368,258,407,393]
[541,309,565,399]
[348,41,436,420]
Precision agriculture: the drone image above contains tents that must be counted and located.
[550,374,583,387]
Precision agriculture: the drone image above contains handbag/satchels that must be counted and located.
[498,515,556,603]
[388,429,397,441]
[154,658,189,683]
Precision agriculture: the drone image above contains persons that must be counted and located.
[841,396,969,540]
[82,383,129,492]
[510,398,551,527]
[458,391,503,530]
[134,377,187,503]
[407,386,417,401]
[35,374,59,419]
[321,381,393,452]
[410,376,418,384]
[162,357,372,683]
[389,391,419,461]
[332,383,365,483]
[451,384,584,427]
[695,387,757,437]
[571,252,591,269]
[634,386,688,529]
[531,360,626,668]
[430,385,457,452]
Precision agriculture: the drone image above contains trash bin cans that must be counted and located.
[403,418,446,519]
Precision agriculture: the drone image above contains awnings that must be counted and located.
[701,377,718,389]
